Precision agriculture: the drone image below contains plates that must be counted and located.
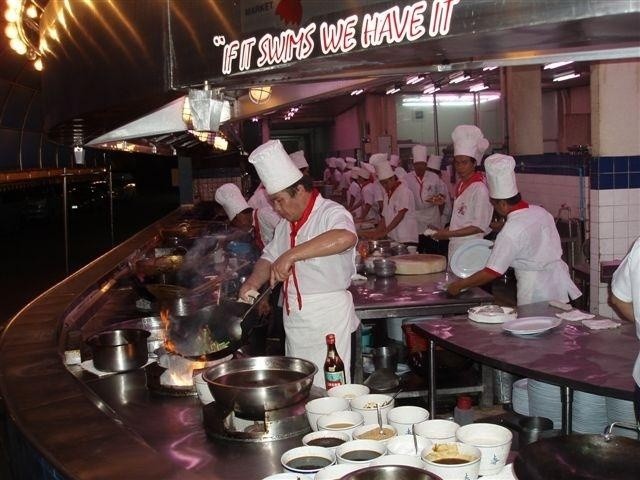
[511,376,638,439]
[501,316,564,336]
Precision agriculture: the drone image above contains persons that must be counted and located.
[447,154,583,306]
[429,125,492,294]
[239,138,362,390]
[215,183,282,257]
[248,144,452,255]
[609,236,640,421]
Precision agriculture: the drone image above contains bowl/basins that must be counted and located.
[200,356,319,418]
[258,383,515,478]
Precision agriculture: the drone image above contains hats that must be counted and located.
[215,182,250,221]
[326,146,443,181]
[485,154,518,200]
[474,138,490,166]
[248,140,303,195]
[289,151,308,169]
[452,125,484,166]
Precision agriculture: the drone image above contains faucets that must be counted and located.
[554,199,573,240]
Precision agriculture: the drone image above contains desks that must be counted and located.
[412,300,640,435]
[347,270,493,409]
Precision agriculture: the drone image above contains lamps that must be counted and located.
[248,86,273,106]
[284,61,581,121]
[182,96,229,151]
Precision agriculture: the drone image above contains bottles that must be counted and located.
[323,333,346,390]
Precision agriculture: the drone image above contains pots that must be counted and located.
[163,299,264,360]
[87,328,151,372]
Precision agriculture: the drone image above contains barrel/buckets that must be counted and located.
[371,345,399,372]
